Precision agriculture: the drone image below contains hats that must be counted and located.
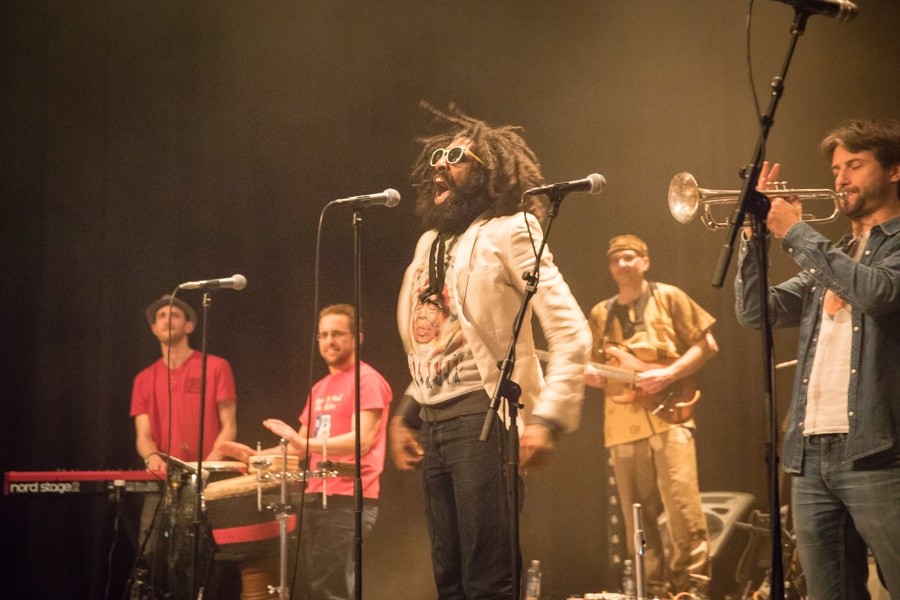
[145,294,198,329]
[607,234,649,257]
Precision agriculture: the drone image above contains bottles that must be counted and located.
[622,559,638,600]
[524,560,542,600]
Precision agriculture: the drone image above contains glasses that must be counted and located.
[430,147,485,167]
[315,329,355,342]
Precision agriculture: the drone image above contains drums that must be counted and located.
[170,455,248,543]
[201,472,300,600]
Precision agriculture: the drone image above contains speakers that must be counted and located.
[657,492,761,600]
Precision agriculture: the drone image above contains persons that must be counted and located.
[129,294,237,554]
[733,116,900,600]
[218,304,392,600]
[584,235,719,600]
[388,102,594,600]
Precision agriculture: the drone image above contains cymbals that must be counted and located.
[774,358,798,371]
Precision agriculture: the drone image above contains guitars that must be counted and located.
[734,396,795,592]
[532,346,699,423]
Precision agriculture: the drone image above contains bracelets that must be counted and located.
[143,452,158,465]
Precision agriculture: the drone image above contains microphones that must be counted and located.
[180,274,248,291]
[780,0,859,22]
[525,173,607,196]
[158,452,211,479]
[332,188,401,208]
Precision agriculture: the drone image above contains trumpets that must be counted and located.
[666,171,851,231]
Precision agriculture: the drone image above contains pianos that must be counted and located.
[3,469,188,600]
